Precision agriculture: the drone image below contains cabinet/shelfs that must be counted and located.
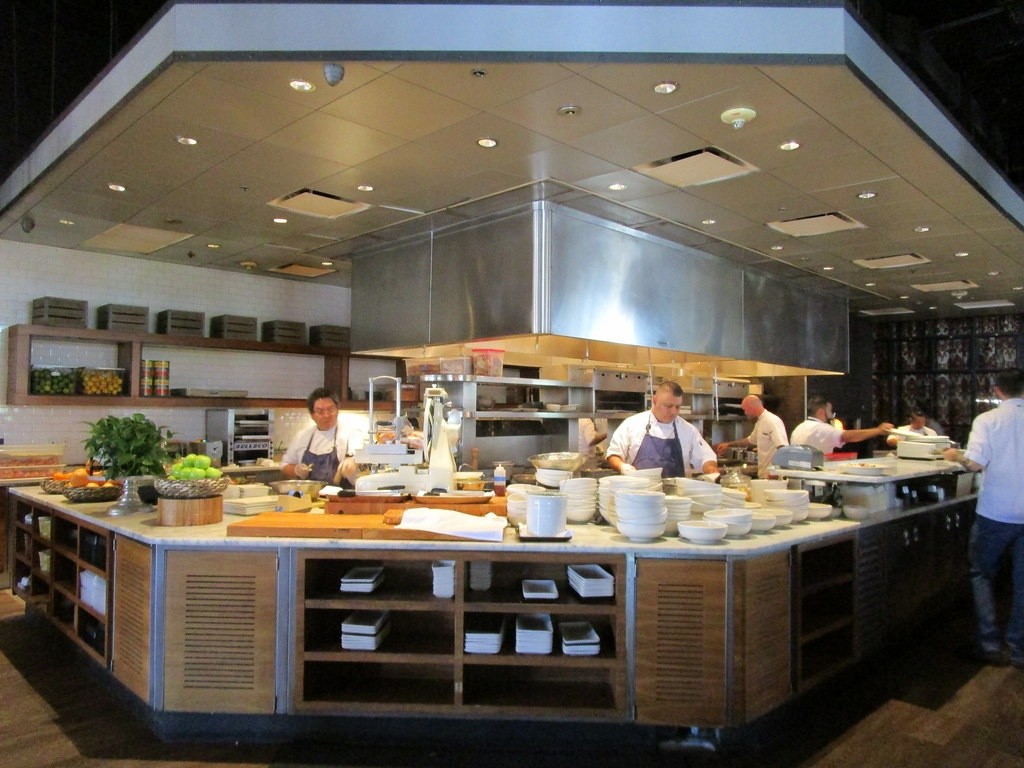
[2,488,986,730]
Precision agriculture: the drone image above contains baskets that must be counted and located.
[153,475,229,498]
[60,480,123,502]
[39,477,71,494]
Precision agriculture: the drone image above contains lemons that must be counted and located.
[82,370,124,396]
[31,368,80,394]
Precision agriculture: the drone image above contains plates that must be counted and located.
[432,560,455,598]
[885,429,923,437]
[62,487,123,502]
[153,478,230,499]
[340,567,386,594]
[40,478,70,494]
[411,495,493,504]
[325,495,408,502]
[457,472,483,479]
[837,463,895,475]
[341,611,391,650]
[464,561,616,655]
[223,485,279,515]
[515,527,572,542]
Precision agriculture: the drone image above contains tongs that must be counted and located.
[337,489,398,497]
[423,487,447,496]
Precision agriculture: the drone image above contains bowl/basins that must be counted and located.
[274,448,287,463]
[269,480,328,502]
[546,404,583,411]
[506,452,868,544]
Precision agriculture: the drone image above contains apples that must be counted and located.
[167,454,221,480]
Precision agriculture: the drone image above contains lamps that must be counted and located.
[322,61,345,90]
[21,218,35,233]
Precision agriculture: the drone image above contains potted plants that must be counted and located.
[80,414,170,517]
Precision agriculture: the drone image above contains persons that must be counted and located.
[944,368,1024,667]
[279,388,363,487]
[790,396,894,453]
[605,381,719,479]
[887,410,937,446]
[579,418,607,458]
[717,395,788,480]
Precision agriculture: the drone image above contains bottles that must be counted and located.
[493,464,506,496]
[720,463,752,502]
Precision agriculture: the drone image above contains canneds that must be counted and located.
[140,360,170,397]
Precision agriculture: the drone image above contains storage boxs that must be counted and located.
[210,314,258,338]
[0,444,65,468]
[157,309,204,336]
[96,304,151,333]
[403,356,439,383]
[472,347,505,378]
[0,464,66,480]
[261,320,307,344]
[310,325,352,349]
[384,383,418,401]
[438,355,470,375]
[29,365,80,395]
[79,367,127,396]
[32,296,90,329]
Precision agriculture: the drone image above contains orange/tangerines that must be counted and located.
[52,468,117,488]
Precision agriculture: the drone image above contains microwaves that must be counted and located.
[205,408,275,466]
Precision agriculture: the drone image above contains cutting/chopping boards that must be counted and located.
[324,497,507,516]
[227,512,494,542]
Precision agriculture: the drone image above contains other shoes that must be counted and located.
[977,648,1003,665]
